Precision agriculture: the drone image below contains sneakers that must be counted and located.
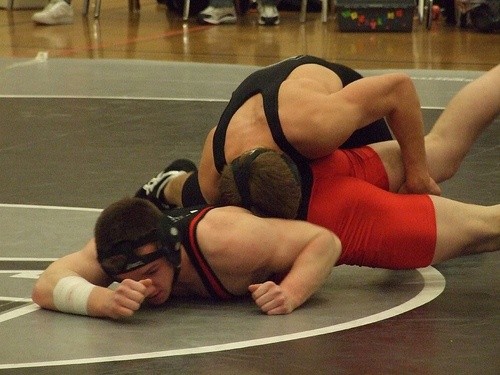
[30,0,74,25]
[198,6,237,24]
[258,3,280,25]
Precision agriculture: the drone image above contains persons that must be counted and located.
[32,0,74,25]
[32,62,500,315]
[134,55,442,219]
[198,0,280,26]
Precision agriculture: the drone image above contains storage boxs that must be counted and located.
[335,0,417,33]
